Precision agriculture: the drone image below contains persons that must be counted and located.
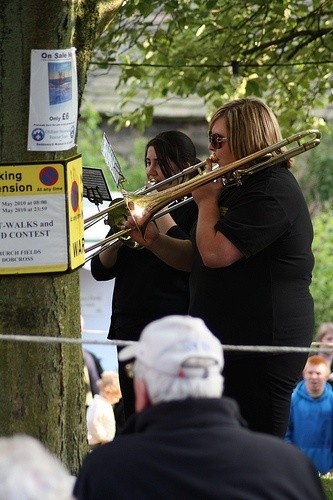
[121,97,316,438]
[92,131,205,422]
[0,315,333,500]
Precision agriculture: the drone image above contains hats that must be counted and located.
[119,314,223,376]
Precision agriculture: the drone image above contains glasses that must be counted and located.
[208,131,228,149]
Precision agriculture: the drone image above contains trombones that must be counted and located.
[81,129,322,254]
[82,164,221,264]
